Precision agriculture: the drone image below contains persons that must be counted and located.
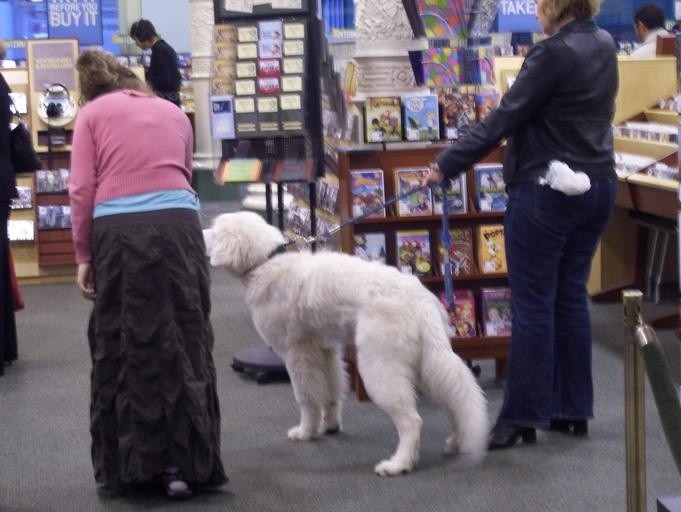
[369,116,388,140]
[418,0,618,450]
[630,4,673,58]
[129,18,182,112]
[271,31,281,39]
[449,303,476,337]
[484,305,512,338]
[66,46,229,498]
[270,45,281,59]
[0,37,24,380]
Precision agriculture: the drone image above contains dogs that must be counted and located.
[202,210,492,479]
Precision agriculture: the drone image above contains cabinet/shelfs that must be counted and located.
[339,148,512,400]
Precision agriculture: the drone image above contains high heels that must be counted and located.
[489,421,535,448]
[550,416,588,434]
